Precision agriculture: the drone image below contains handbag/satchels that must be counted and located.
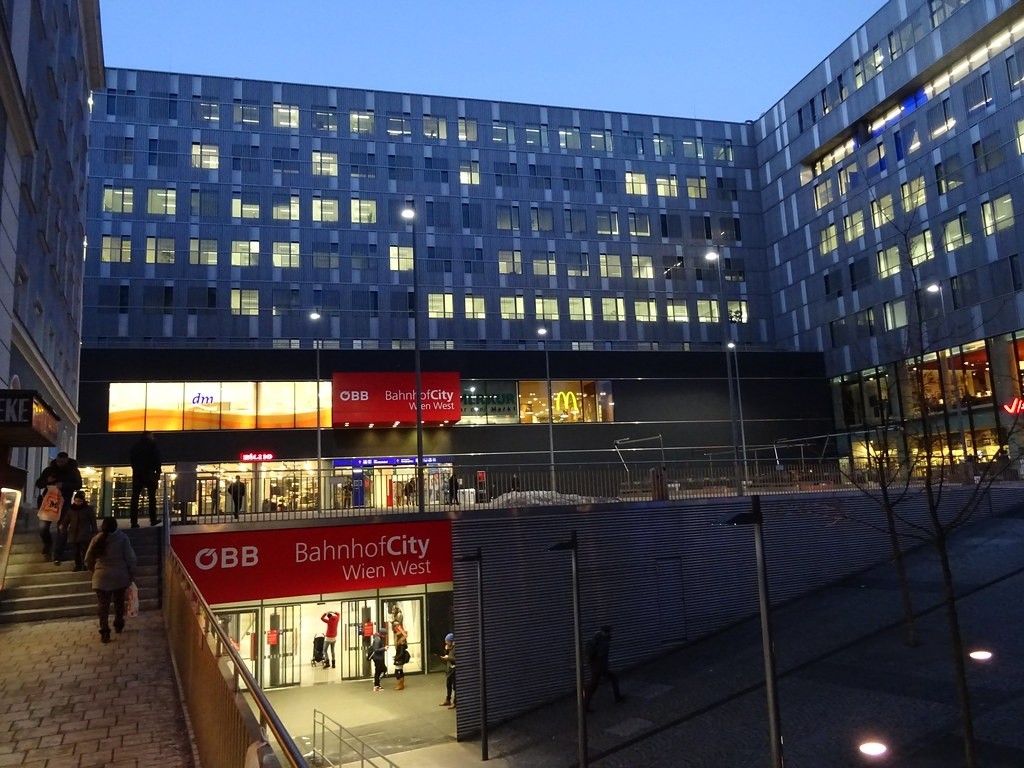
[447,662,455,679]
[404,650,410,663]
[128,582,139,618]
[367,647,376,660]
[37,485,64,522]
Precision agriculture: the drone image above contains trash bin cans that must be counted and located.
[650,466,669,501]
[959,462,975,486]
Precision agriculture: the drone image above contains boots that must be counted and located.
[393,677,404,690]
[324,660,329,668]
[332,660,336,668]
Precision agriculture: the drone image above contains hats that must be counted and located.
[380,628,387,634]
[445,633,454,641]
[74,491,85,501]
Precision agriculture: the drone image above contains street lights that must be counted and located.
[728,340,749,493]
[706,245,744,497]
[723,493,784,768]
[310,307,322,517]
[456,545,488,760]
[928,279,975,485]
[538,323,557,504]
[546,529,590,768]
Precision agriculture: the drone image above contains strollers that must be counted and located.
[311,633,325,666]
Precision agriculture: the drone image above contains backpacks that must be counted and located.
[585,638,596,656]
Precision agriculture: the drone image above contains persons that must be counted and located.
[584,626,629,714]
[372,628,389,692]
[85,517,137,643]
[440,633,455,710]
[58,491,97,572]
[449,474,461,505]
[405,480,415,506]
[228,475,245,518]
[321,611,340,669]
[392,622,408,690]
[343,482,353,509]
[36,452,83,564]
[390,606,403,659]
[210,487,221,515]
[130,430,162,529]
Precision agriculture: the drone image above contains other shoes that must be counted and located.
[448,703,456,709]
[73,566,81,571]
[151,519,162,525]
[374,686,384,691]
[115,626,123,632]
[42,546,49,556]
[54,558,60,563]
[439,700,451,705]
[583,709,594,714]
[131,524,140,528]
[615,697,626,706]
[101,633,110,641]
